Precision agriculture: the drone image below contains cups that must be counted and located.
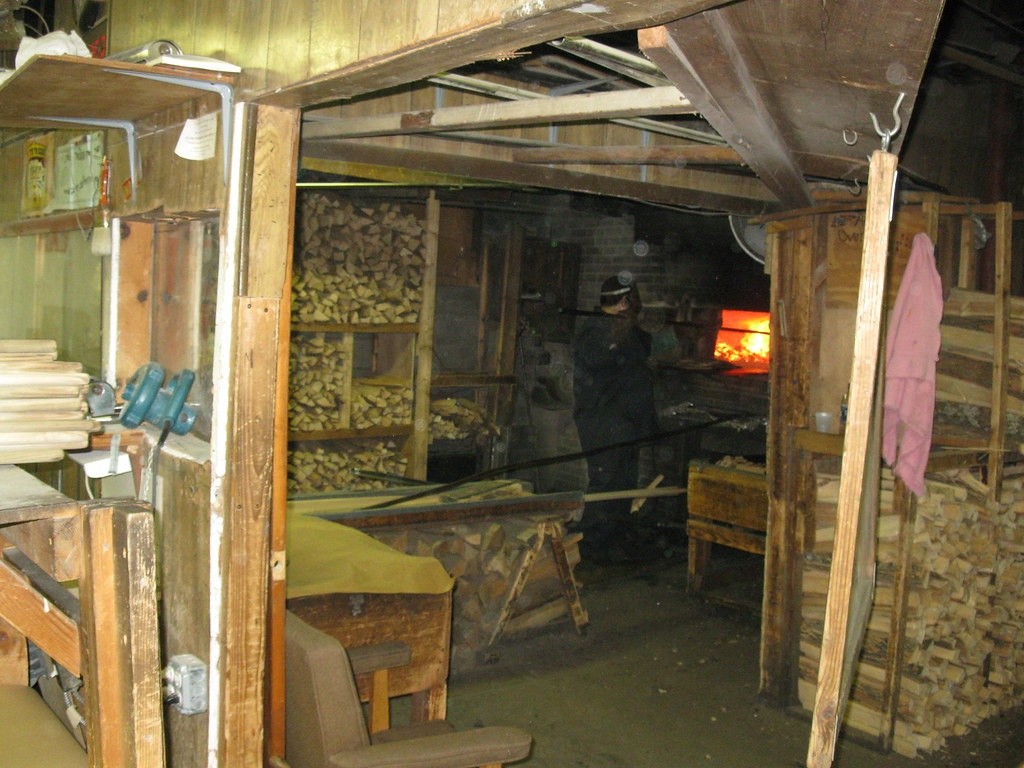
[815,412,832,433]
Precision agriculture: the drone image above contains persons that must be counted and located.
[573,276,670,565]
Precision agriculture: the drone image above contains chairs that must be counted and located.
[285,608,532,768]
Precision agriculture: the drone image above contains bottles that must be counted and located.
[840,383,850,426]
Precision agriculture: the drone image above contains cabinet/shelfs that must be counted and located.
[752,195,1020,754]
[288,188,517,494]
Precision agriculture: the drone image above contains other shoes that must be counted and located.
[574,536,643,566]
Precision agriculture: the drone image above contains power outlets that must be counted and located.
[162,653,209,716]
